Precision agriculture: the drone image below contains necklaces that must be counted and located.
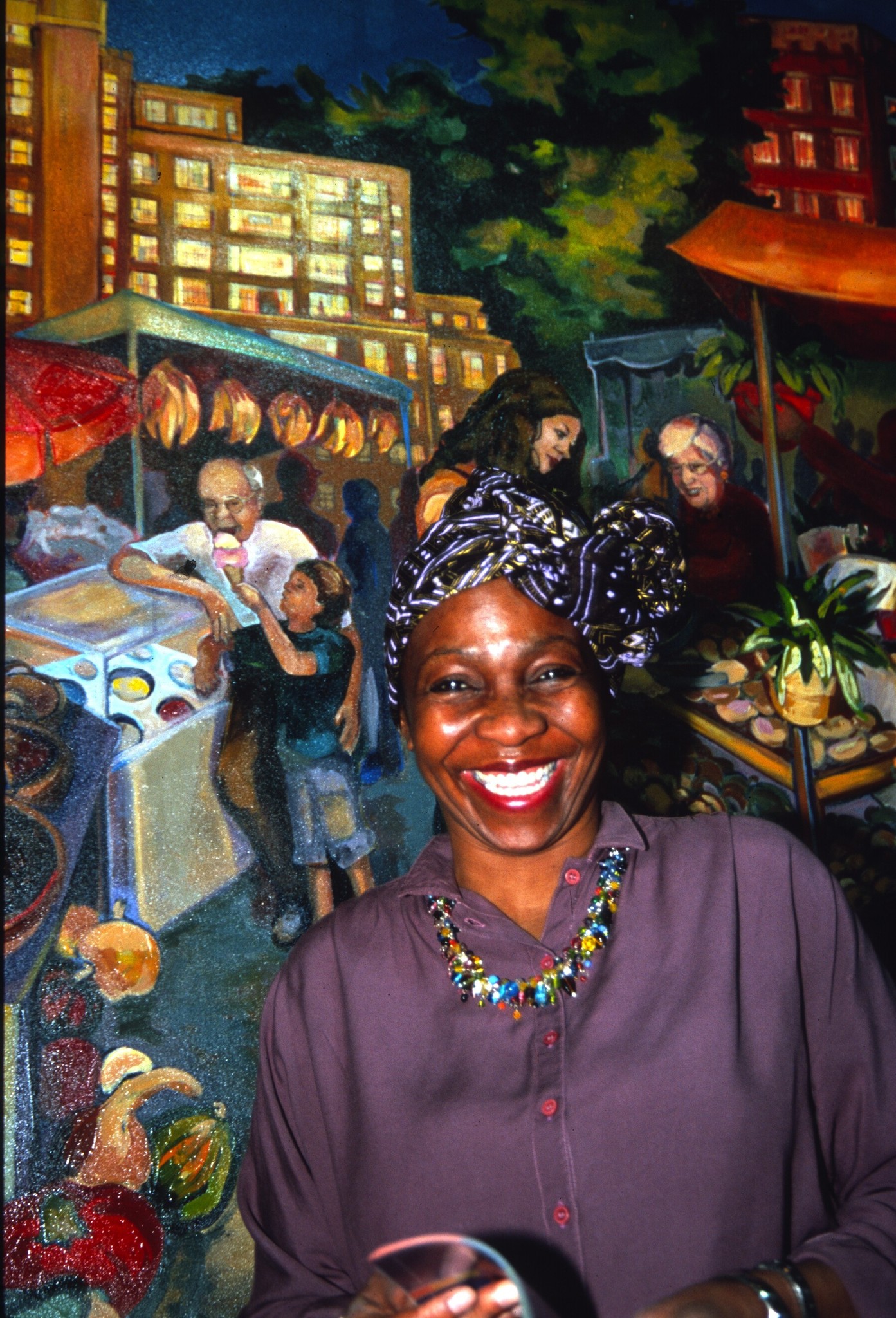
[425,843,626,1013]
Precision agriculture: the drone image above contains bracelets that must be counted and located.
[719,1258,818,1318]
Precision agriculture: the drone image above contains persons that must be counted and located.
[252,463,896,1318]
